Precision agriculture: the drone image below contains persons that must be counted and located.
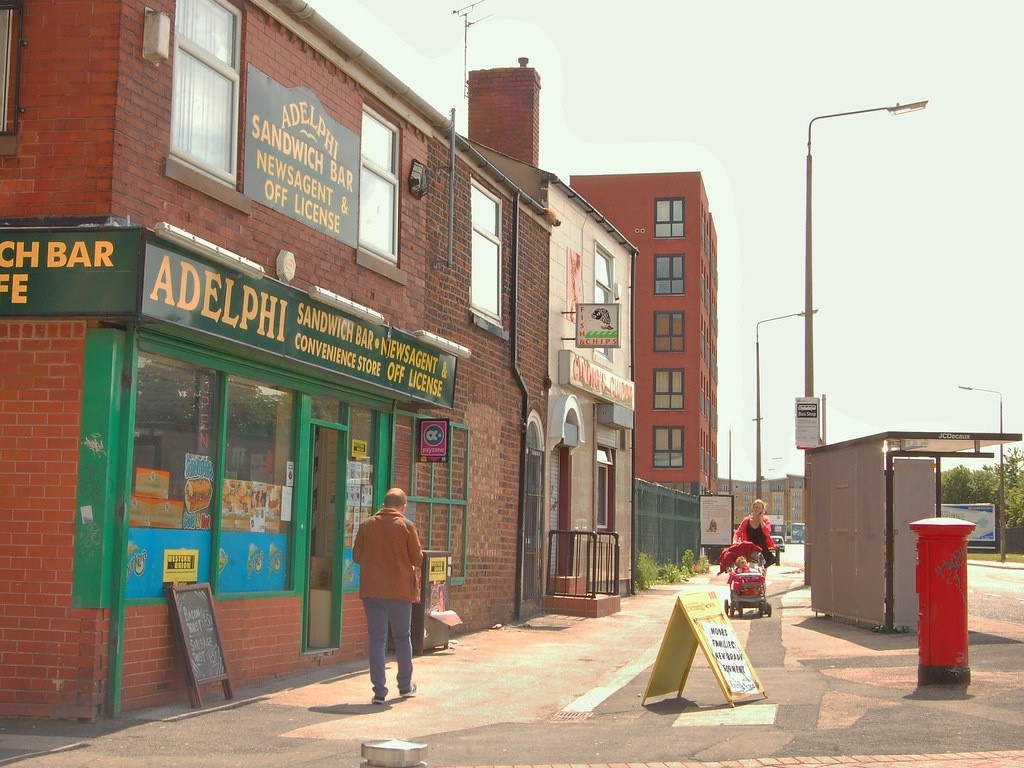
[734,556,759,575]
[352,488,423,704]
[733,499,777,551]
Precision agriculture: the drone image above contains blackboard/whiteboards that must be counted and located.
[170,582,228,685]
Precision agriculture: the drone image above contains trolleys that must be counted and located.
[724,543,772,618]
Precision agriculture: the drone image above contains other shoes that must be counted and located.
[372,696,385,703]
[400,685,418,696]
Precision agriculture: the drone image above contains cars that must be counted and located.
[770,536,785,552]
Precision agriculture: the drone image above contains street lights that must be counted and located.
[804,98,928,399]
[958,385,1006,562]
[756,309,818,500]
